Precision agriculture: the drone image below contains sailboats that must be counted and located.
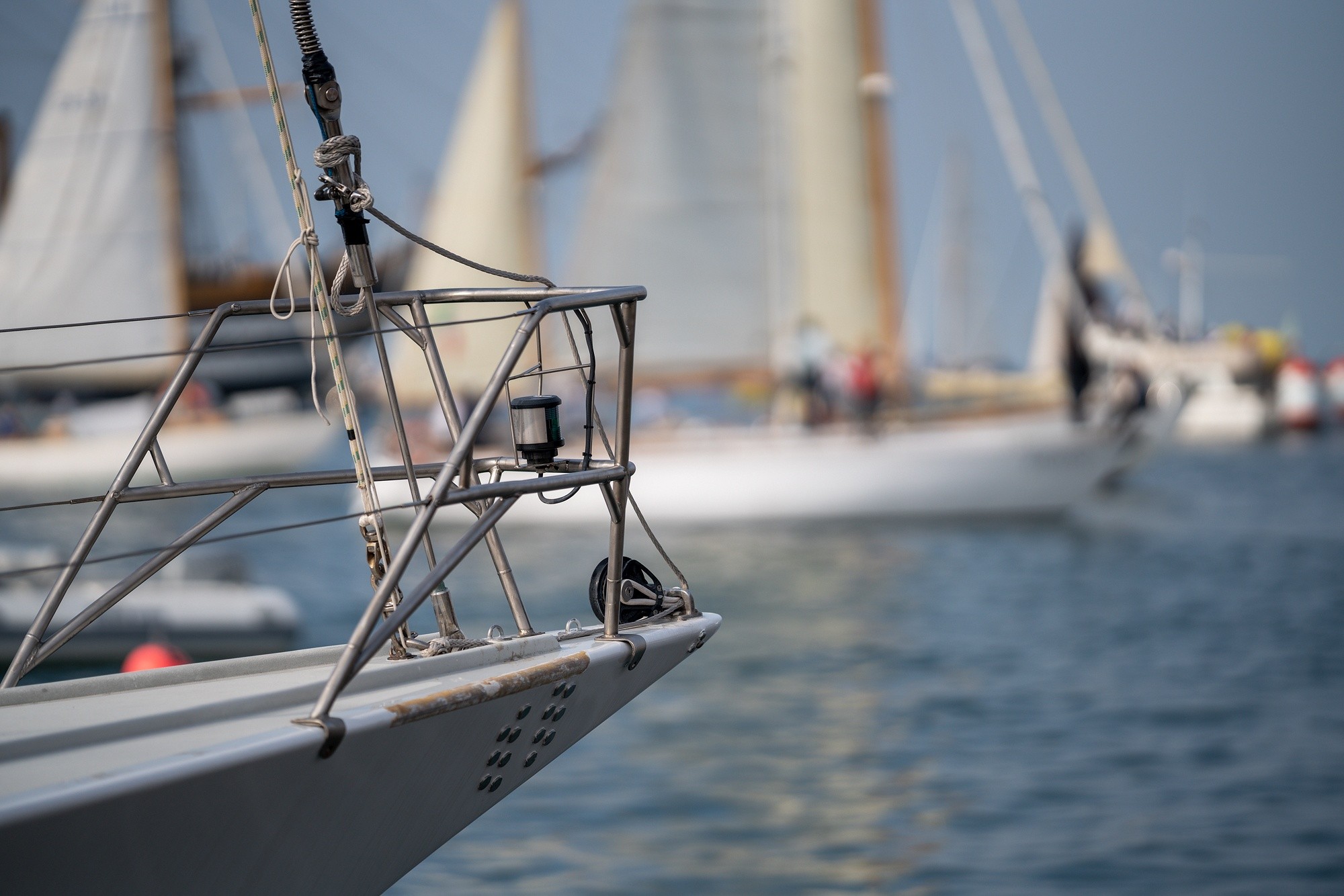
[363,2,1343,528]
[0,0,419,499]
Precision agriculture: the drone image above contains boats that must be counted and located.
[0,286,723,896]
